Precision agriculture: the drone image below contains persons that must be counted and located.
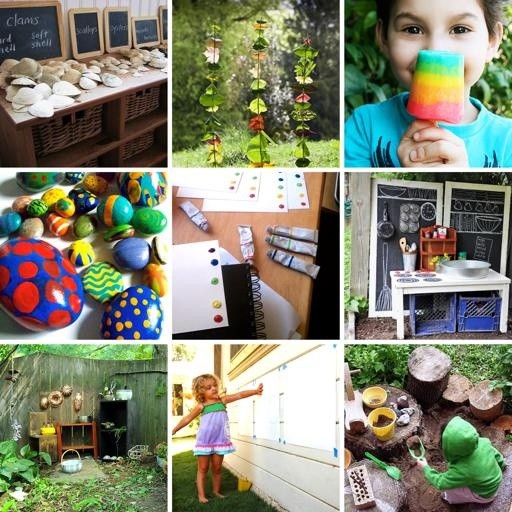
[345,1,511,168]
[414,415,509,507]
[405,435,426,460]
[171,372,267,500]
[363,450,401,481]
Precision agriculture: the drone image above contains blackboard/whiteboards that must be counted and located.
[105,8,132,53]
[370,182,509,318]
[132,16,160,49]
[0,0,68,65]
[159,5,167,44]
[69,7,105,60]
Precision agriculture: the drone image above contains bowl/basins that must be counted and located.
[78,416,91,423]
[438,259,492,281]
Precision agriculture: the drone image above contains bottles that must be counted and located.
[432,225,437,239]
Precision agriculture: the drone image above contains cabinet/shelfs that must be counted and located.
[59,399,128,463]
[420,226,458,271]
[0,72,163,167]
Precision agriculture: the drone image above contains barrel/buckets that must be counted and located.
[368,407,396,440]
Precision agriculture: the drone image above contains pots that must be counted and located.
[376,199,395,240]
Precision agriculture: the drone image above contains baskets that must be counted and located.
[128,445,149,460]
[61,449,83,473]
[409,293,501,336]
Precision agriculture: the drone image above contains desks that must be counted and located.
[172,170,324,341]
[390,265,511,338]
